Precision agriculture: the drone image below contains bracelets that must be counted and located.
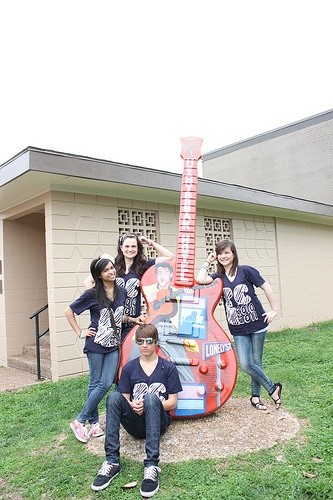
[148,240,154,246]
[205,260,210,266]
[79,330,82,338]
[202,266,208,272]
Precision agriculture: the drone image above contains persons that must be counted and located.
[65,258,143,440]
[90,324,182,497]
[83,233,175,343]
[195,239,282,412]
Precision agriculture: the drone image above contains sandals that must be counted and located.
[250,396,267,410]
[269,382,281,409]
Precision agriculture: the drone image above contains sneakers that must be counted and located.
[70,420,90,442]
[139,465,162,497]
[91,461,122,490]
[88,422,104,436]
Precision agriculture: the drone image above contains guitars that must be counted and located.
[117,136,237,420]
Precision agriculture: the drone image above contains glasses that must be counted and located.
[135,339,156,345]
